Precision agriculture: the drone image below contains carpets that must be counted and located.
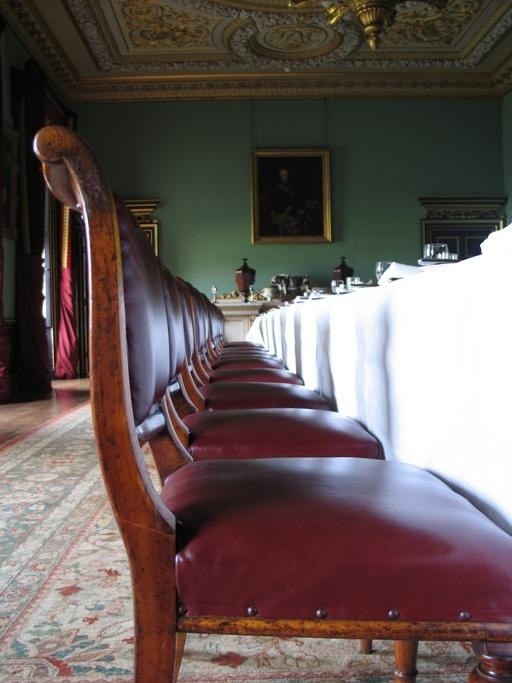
[0,391,477,683]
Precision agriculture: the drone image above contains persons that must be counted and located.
[266,165,297,235]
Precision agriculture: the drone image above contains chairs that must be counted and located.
[30,125,512,683]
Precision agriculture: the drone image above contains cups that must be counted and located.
[424,243,449,260]
[331,279,344,293]
[346,276,360,289]
[375,261,391,280]
